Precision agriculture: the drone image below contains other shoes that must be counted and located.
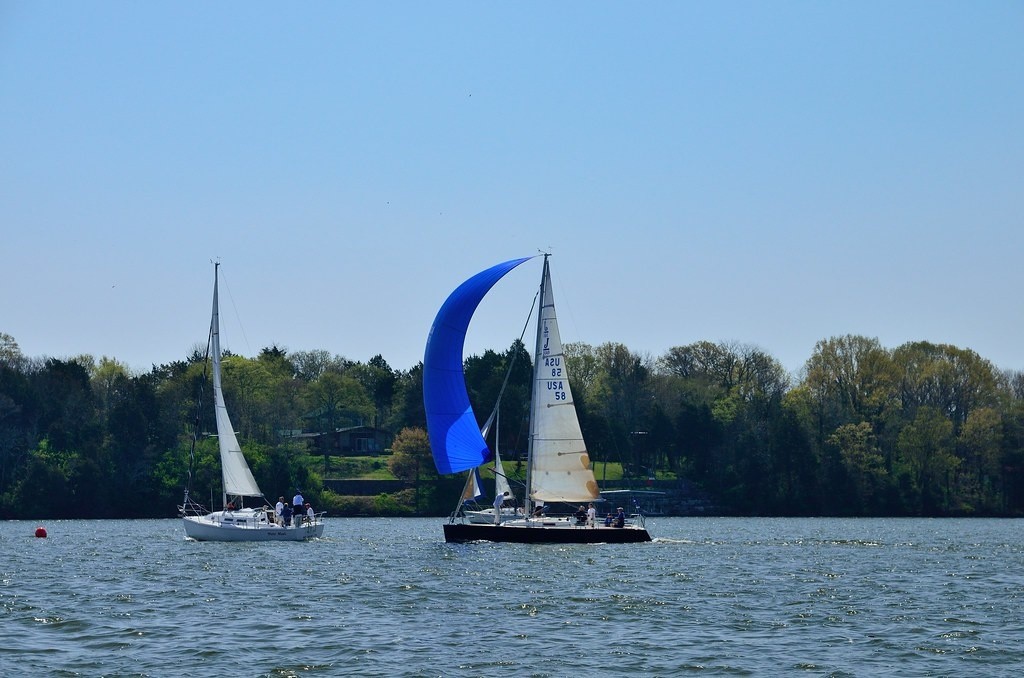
[495,522,500,526]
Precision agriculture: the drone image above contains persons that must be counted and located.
[492,491,508,523]
[587,503,595,526]
[605,513,612,527]
[305,503,314,523]
[276,497,285,528]
[572,505,587,525]
[616,507,625,528]
[278,503,293,525]
[293,491,304,514]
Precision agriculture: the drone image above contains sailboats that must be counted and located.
[421,249,653,543]
[176,259,327,541]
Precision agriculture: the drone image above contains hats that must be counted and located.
[305,503,310,506]
[617,507,623,510]
